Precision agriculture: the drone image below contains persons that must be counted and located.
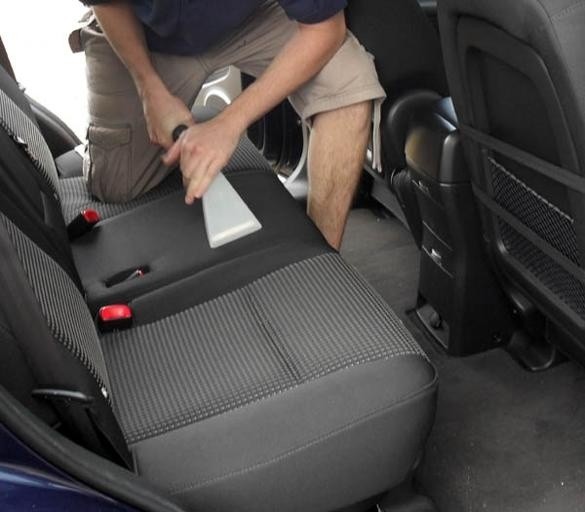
[69,1,386,253]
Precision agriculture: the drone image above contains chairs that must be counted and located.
[437,0,585,370]
